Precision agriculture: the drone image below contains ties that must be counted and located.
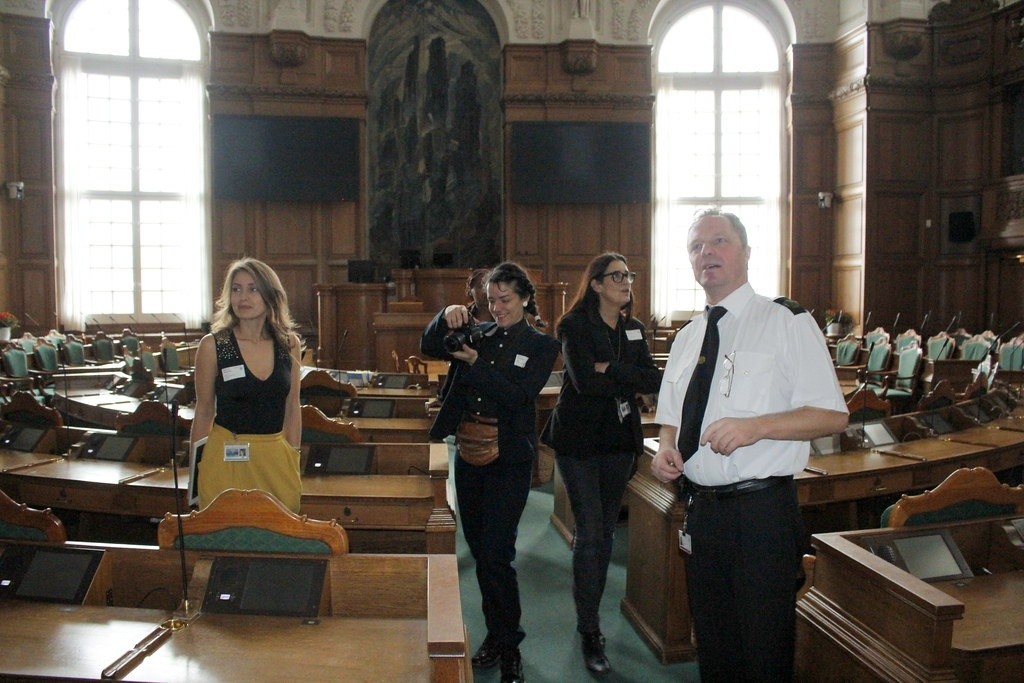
[677,305,727,463]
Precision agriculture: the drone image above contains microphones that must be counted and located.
[307,320,317,369]
[635,310,695,364]
[810,309,1024,451]
[170,399,189,613]
[23,313,191,470]
[338,330,349,417]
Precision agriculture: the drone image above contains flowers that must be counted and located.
[0,311,20,329]
[825,308,842,324]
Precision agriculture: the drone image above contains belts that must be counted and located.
[691,475,793,503]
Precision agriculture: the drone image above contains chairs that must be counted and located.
[832,327,1024,425]
[881,467,1024,528]
[0,329,430,553]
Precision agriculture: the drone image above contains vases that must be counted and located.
[0,327,11,340]
[826,323,839,336]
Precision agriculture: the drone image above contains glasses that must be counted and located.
[597,271,636,284]
[719,350,737,398]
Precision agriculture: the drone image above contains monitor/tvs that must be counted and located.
[890,529,974,584]
[966,404,992,423]
[862,420,900,448]
[923,413,954,434]
[8,374,410,618]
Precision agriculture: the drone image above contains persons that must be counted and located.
[467,269,494,322]
[651,213,850,683]
[418,263,557,682]
[189,259,303,516]
[539,254,658,675]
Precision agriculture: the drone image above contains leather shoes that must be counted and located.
[582,630,612,676]
[472,635,502,667]
[500,645,524,683]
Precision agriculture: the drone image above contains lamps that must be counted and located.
[6,181,24,199]
[818,192,834,209]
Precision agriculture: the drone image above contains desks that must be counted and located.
[0,346,474,683]
[620,405,1024,683]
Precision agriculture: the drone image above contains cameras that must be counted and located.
[442,322,484,353]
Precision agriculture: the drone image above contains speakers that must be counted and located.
[945,211,977,242]
